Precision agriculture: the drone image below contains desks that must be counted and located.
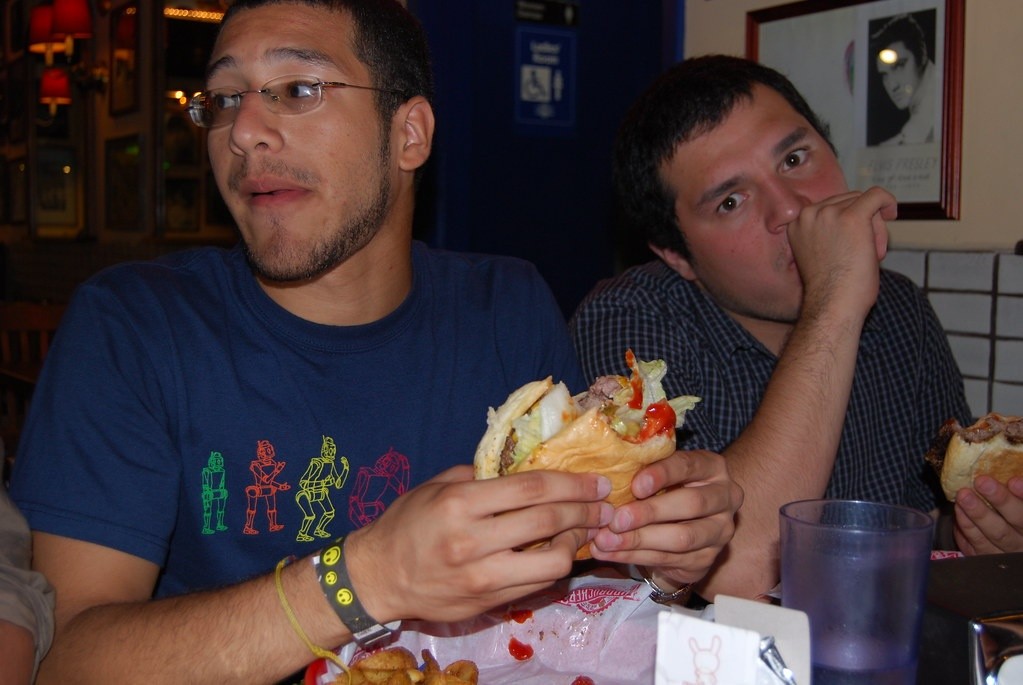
[916,552,1023,685]
[0,360,48,400]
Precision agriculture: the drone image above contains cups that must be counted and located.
[780,496,936,685]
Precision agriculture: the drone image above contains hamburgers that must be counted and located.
[473,349,702,559]
[926,411,1022,510]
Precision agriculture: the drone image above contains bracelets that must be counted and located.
[315,534,401,648]
[629,565,696,604]
[275,555,351,678]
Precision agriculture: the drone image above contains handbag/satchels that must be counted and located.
[917,550,1023,685]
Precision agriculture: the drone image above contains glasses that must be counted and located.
[184,75,409,129]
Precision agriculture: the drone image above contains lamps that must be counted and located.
[27,0,94,115]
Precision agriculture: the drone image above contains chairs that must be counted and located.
[1,301,70,468]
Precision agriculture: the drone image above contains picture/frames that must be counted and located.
[745,0,968,219]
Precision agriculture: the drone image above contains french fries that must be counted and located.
[321,644,479,685]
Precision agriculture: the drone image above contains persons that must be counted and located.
[568,52,1023,606]
[0,0,745,685]
[870,14,938,146]
